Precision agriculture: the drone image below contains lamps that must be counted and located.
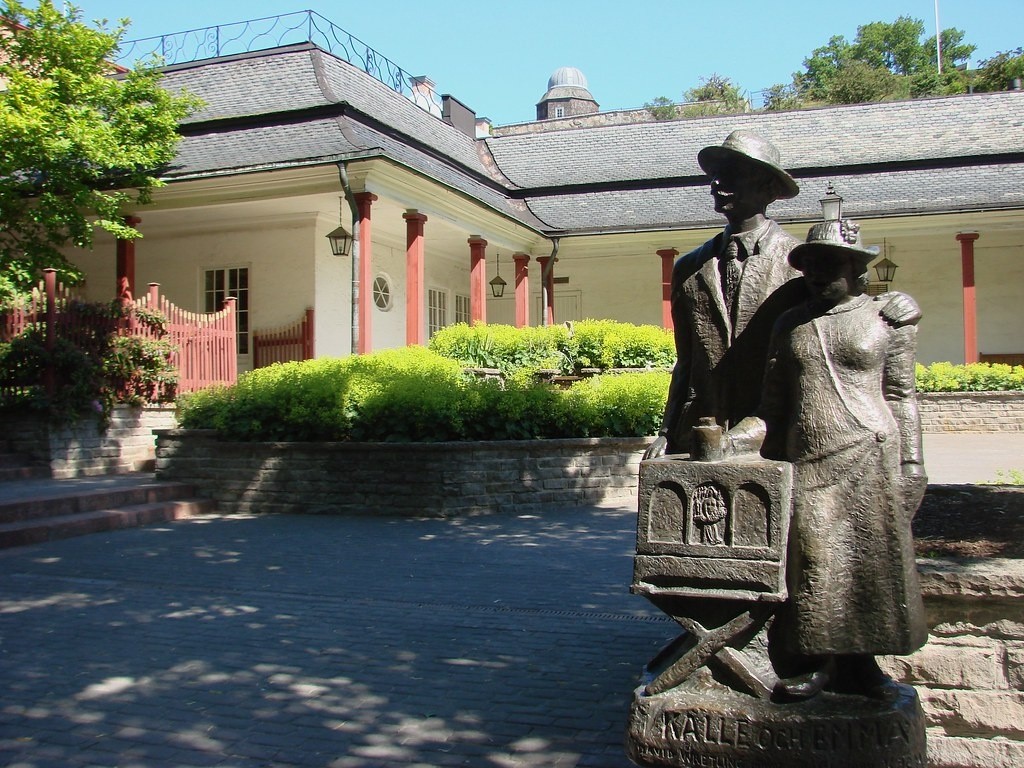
[872,237,899,281]
[325,196,352,256]
[489,254,507,297]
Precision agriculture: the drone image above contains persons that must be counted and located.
[641,130,924,460]
[720,219,930,704]
